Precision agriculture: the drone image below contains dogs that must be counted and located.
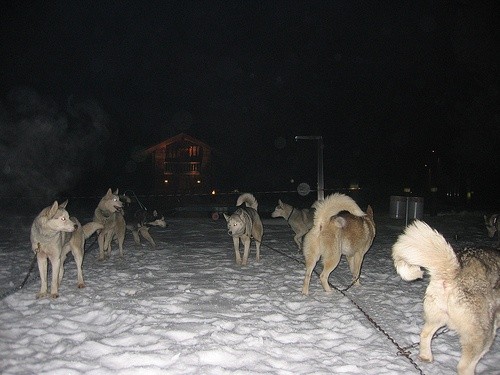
[271,199,316,251]
[93,186,126,261]
[303,191,377,296]
[29,197,105,299]
[222,192,265,267]
[126,196,170,246]
[392,218,500,374]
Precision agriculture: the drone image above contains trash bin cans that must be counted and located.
[409,195,424,221]
[389,195,406,219]
